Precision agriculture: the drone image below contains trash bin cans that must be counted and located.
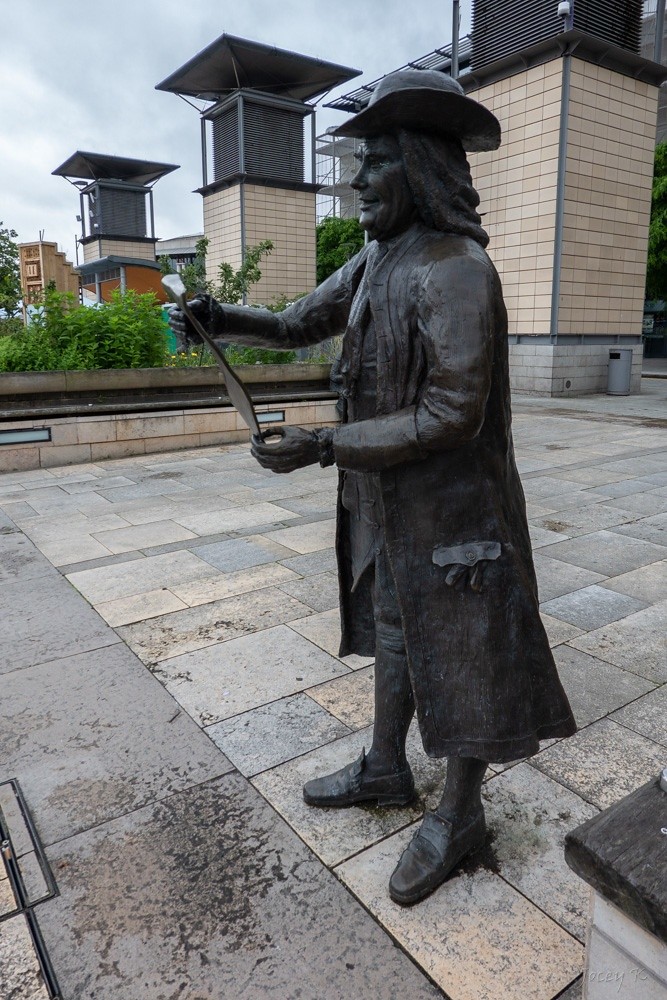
[607,348,633,396]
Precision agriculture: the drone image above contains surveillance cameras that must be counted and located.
[558,2,570,20]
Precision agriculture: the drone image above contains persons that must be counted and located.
[167,71,580,908]
